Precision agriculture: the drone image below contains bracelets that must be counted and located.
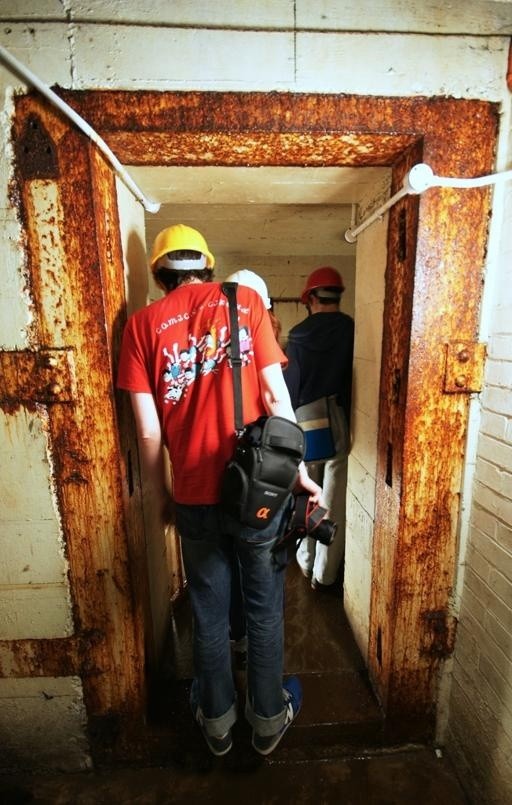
[271,322,282,332]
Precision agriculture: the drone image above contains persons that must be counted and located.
[160,326,253,385]
[222,270,295,681]
[115,223,330,758]
[282,267,356,590]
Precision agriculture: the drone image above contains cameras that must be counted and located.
[289,500,338,546]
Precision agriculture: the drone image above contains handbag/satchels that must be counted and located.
[218,412,304,530]
[286,394,350,463]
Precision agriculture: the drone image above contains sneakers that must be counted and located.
[190,675,303,756]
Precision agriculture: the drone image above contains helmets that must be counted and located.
[298,267,347,303]
[225,269,273,311]
[150,223,216,275]
